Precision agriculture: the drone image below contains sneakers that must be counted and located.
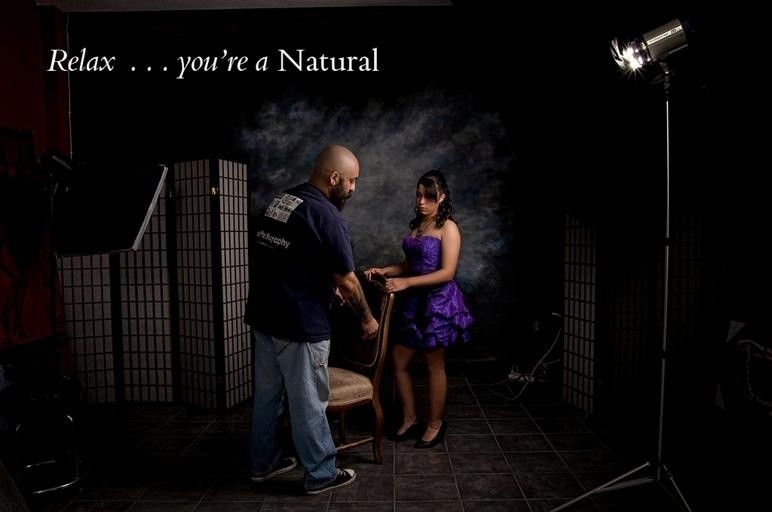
[304,467,357,497]
[249,455,298,483]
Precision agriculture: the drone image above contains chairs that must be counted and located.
[271,268,394,469]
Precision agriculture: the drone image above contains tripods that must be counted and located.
[549,76,692,512]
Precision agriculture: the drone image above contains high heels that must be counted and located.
[413,418,447,450]
[392,416,423,443]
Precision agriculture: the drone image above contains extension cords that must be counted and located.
[508,372,535,382]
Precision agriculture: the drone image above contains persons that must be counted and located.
[244,144,379,495]
[364,170,473,448]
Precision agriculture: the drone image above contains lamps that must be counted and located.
[17,146,170,495]
[534,5,711,505]
